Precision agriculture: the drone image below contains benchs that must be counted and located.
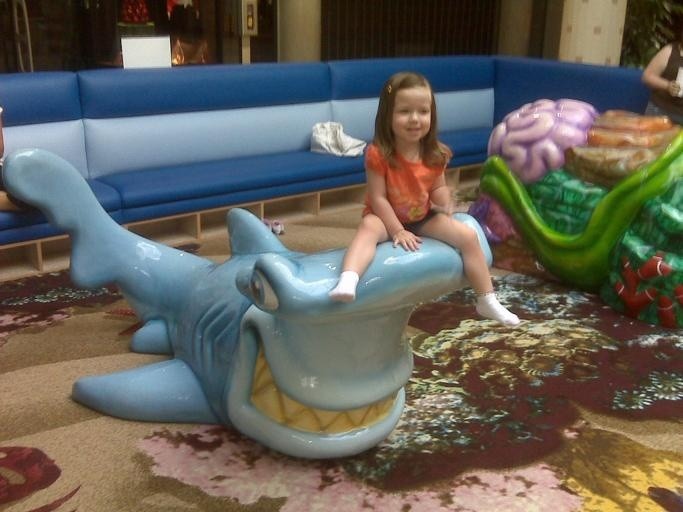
[1,58,652,283]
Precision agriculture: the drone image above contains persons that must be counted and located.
[639,9,683,129]
[328,71,522,328]
